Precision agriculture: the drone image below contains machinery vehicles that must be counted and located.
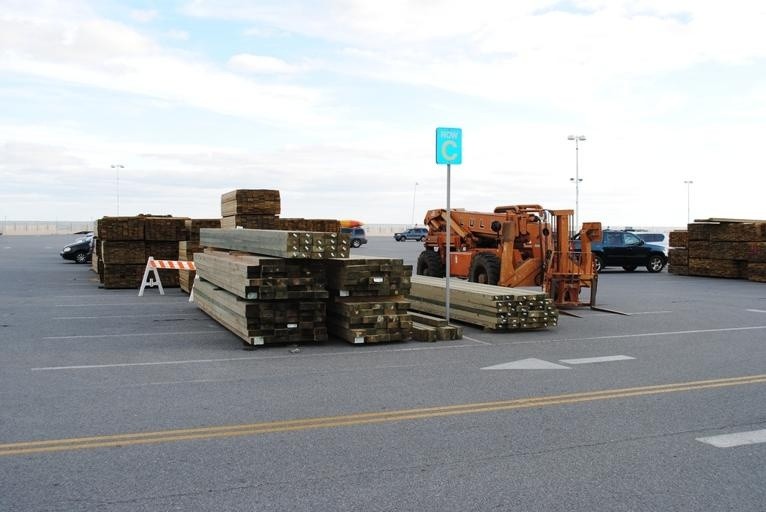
[409,199,633,317]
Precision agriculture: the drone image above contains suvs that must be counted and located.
[339,226,368,249]
[393,226,429,242]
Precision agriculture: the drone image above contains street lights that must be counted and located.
[410,182,421,223]
[682,180,694,223]
[567,135,587,235]
[109,164,126,217]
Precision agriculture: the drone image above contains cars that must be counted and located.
[58,229,92,265]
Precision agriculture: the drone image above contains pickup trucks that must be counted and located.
[564,226,668,275]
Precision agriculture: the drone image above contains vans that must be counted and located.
[627,230,669,256]
